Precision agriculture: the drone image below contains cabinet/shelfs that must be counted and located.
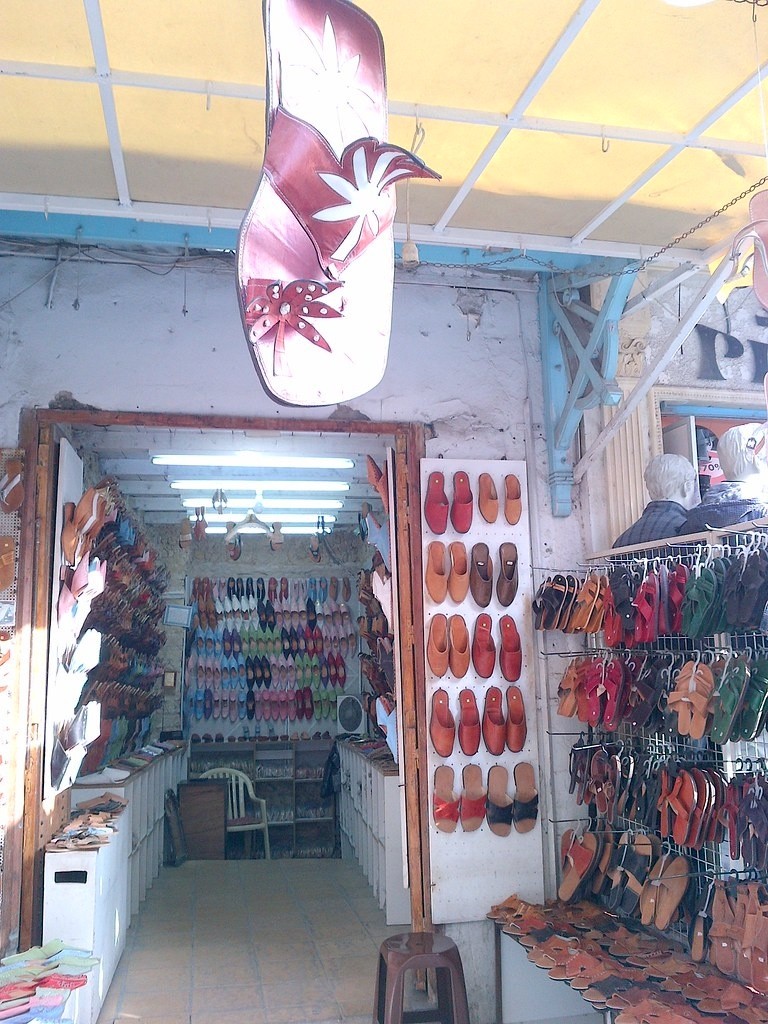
[486,517,768,1024]
[42,742,412,1024]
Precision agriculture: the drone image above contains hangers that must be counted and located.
[223,507,275,544]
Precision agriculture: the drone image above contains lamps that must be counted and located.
[150,454,356,534]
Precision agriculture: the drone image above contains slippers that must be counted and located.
[447,541,469,603]
[430,689,456,758]
[234,0,442,406]
[478,472,499,523]
[39,487,185,854]
[424,472,449,535]
[724,188,768,310]
[433,768,460,834]
[458,689,481,756]
[448,615,471,679]
[366,454,389,516]
[473,542,768,1024]
[462,765,487,832]
[504,474,521,526]
[0,938,99,1024]
[426,614,449,677]
[449,470,473,534]
[425,540,450,602]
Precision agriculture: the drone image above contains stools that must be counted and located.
[372,931,471,1024]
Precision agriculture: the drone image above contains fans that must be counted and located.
[336,694,367,736]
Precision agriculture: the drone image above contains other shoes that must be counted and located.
[183,481,398,861]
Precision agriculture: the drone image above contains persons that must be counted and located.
[611,454,696,567]
[678,423,768,540]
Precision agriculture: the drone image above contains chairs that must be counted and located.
[199,768,271,860]
